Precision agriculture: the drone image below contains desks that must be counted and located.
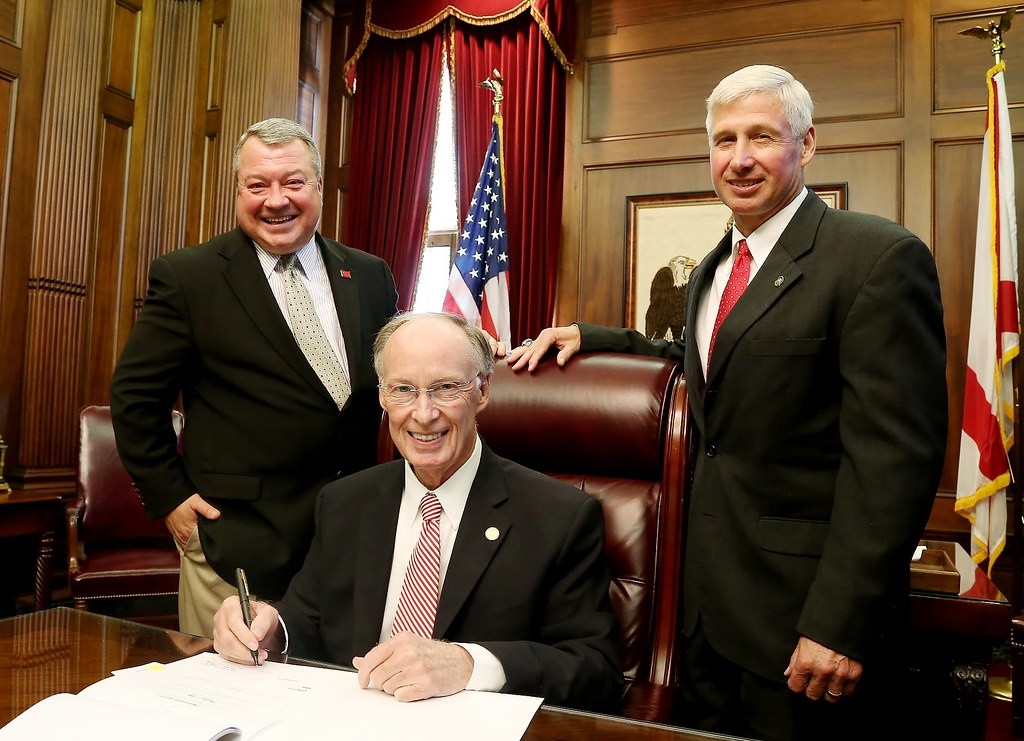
[0,605,757,741]
[904,538,1013,640]
[0,489,63,612]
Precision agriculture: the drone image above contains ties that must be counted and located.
[273,254,351,412]
[707,239,752,375]
[390,493,443,641]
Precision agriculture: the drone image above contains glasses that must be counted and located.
[377,372,479,406]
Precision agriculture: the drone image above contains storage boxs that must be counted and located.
[909,549,960,595]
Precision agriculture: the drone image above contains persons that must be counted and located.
[213,314,621,715]
[109,119,507,638]
[505,64,951,741]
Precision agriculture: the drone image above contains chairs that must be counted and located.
[63,404,185,631]
[378,353,696,723]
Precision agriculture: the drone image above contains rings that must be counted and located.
[489,339,494,341]
[828,691,842,698]
[522,339,532,347]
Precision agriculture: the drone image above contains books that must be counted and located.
[0,652,354,741]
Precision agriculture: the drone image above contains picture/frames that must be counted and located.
[620,181,850,343]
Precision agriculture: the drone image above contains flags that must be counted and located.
[954,62,1022,578]
[443,115,511,353]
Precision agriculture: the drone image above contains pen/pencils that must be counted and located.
[235,566,261,670]
